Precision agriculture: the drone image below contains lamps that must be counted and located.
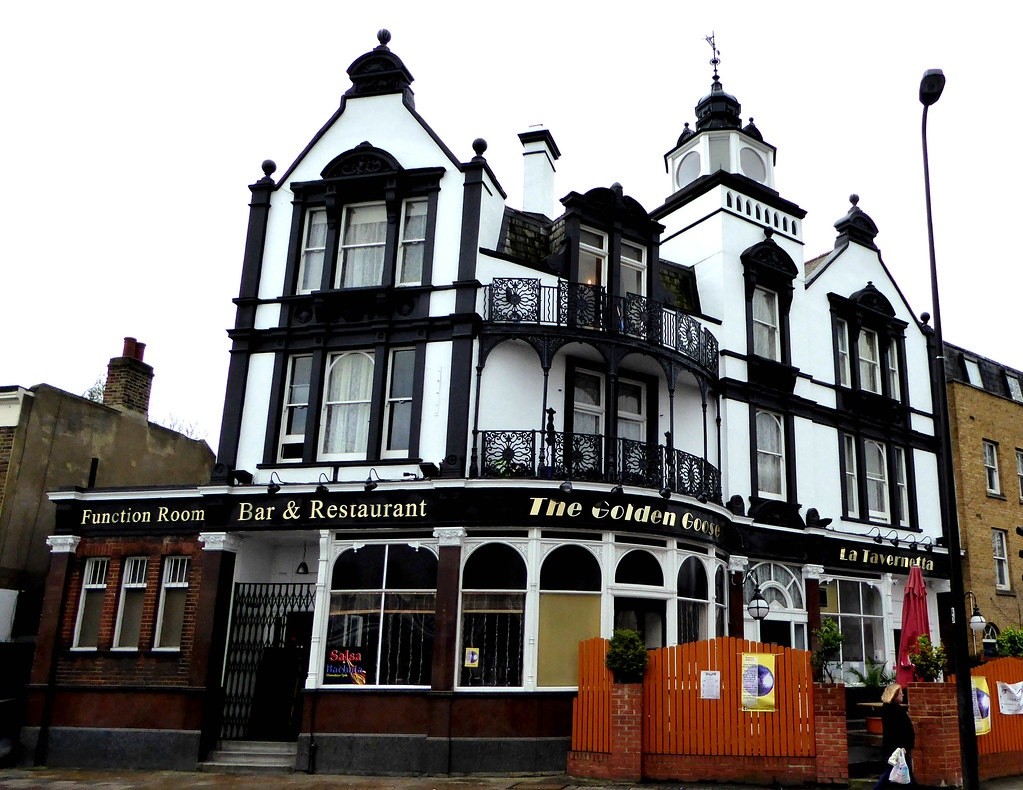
[267,471,287,493]
[560,482,571,494]
[963,591,987,630]
[659,486,671,503]
[883,530,900,547]
[610,484,624,498]
[867,527,884,544]
[364,468,380,491]
[296,541,310,575]
[697,492,708,505]
[315,472,331,494]
[903,534,918,550]
[921,535,934,552]
[730,568,770,620]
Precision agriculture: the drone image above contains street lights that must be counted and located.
[918,67,987,790]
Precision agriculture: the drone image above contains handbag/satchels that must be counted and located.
[887,747,911,784]
[887,748,901,766]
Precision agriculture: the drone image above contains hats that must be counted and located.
[879,684,901,704]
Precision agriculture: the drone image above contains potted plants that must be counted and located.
[605,627,648,684]
[848,654,891,734]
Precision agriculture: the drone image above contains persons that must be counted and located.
[874,684,919,790]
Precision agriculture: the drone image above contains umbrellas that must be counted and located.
[896,564,931,687]
